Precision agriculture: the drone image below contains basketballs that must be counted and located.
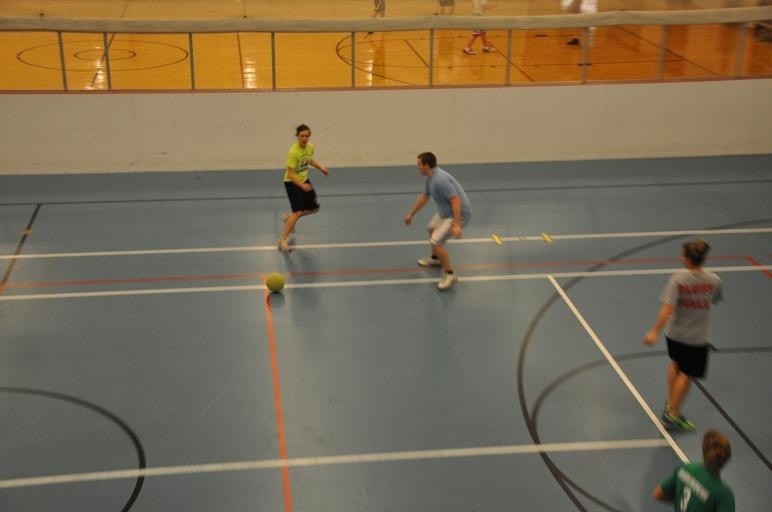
[266,273,285,291]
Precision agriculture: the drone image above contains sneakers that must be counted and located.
[276,239,292,253]
[659,411,697,433]
[437,271,457,291]
[280,214,297,234]
[416,257,442,267]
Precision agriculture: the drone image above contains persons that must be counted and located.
[404,152,472,291]
[651,428,735,510]
[367,0,387,35]
[560,0,581,48]
[575,2,598,66]
[437,0,455,17]
[276,124,330,254]
[463,1,499,54]
[642,240,725,433]
